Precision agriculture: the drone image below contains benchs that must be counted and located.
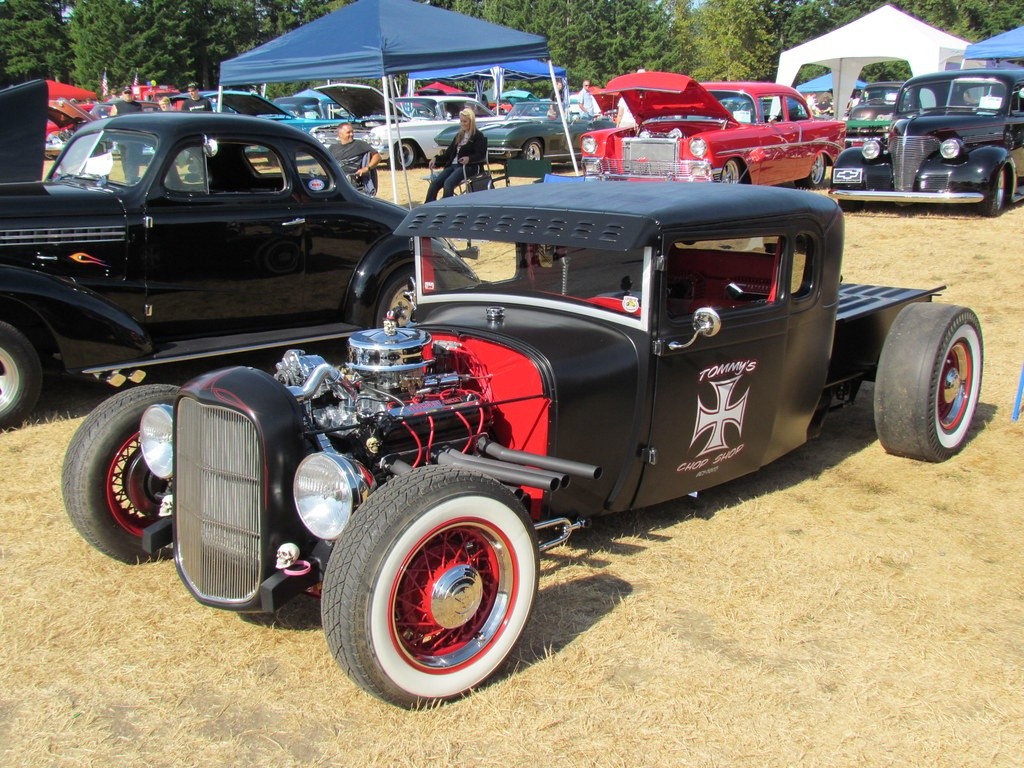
[670,246,782,303]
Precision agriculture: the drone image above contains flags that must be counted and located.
[102,71,109,97]
[133,71,139,86]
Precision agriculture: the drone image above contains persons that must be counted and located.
[107,87,120,102]
[328,122,383,192]
[110,89,142,117]
[69,98,79,104]
[181,81,213,111]
[159,97,171,111]
[423,108,486,205]
[83,97,93,105]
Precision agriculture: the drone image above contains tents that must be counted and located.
[393,82,466,107]
[766,4,972,120]
[405,59,571,123]
[795,72,869,92]
[958,26,1024,69]
[45,79,97,101]
[215,0,580,207]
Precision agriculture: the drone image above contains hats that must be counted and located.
[159,97,170,105]
[122,85,133,92]
[187,83,198,89]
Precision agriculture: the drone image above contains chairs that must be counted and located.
[354,149,380,194]
[487,156,552,184]
[544,172,603,184]
[429,136,493,191]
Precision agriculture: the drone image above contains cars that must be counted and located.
[437,100,621,169]
[310,81,546,167]
[842,81,922,147]
[580,71,849,194]
[41,95,165,162]
[62,176,986,712]
[173,89,359,158]
[830,68,1024,220]
[0,79,479,434]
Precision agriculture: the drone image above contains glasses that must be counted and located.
[124,91,132,94]
[187,88,197,93]
[584,84,590,87]
